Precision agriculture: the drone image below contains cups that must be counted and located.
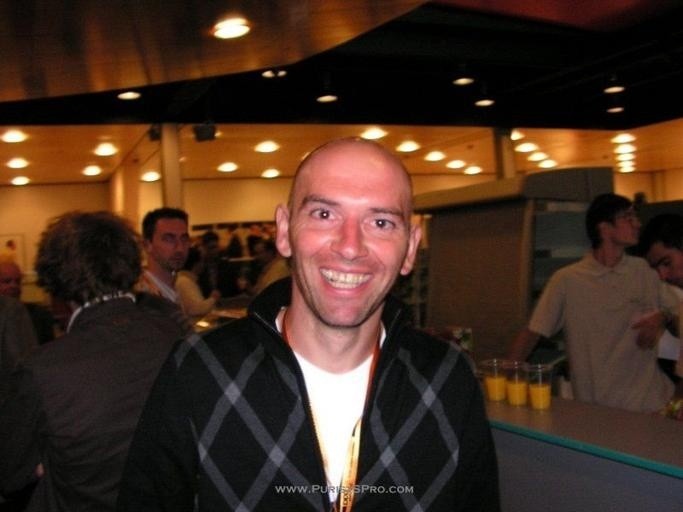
[522,361,553,410]
[479,357,507,403]
[501,359,529,406]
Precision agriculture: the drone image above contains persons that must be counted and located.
[136,207,190,306]
[639,208,683,421]
[0,260,55,346]
[116,134,502,511]
[1,208,185,512]
[493,191,683,416]
[0,294,40,374]
[175,231,293,315]
